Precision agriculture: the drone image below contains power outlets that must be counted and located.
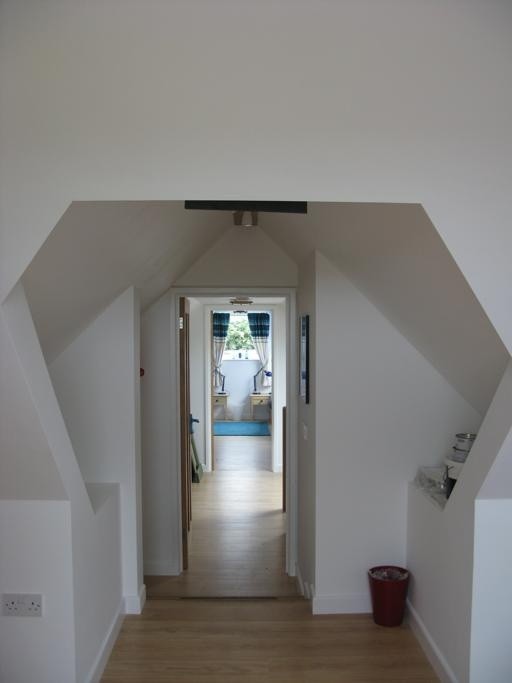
[1,593,42,617]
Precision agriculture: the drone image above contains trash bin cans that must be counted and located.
[368,565,409,626]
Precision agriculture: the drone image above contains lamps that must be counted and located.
[228,296,252,313]
[214,365,227,394]
[235,201,257,232]
[251,366,272,394]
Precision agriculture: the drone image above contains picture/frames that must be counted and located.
[298,314,310,404]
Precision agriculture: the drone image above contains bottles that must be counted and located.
[239,349,248,359]
